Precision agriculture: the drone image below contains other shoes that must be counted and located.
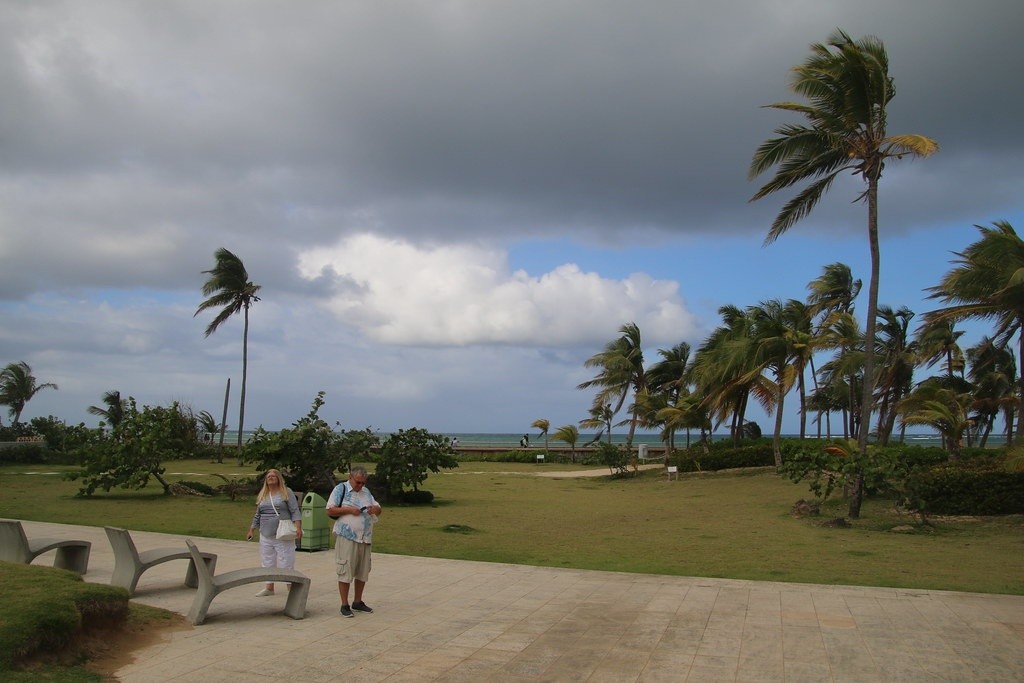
[255,587,275,597]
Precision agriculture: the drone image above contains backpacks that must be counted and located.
[520,439,525,447]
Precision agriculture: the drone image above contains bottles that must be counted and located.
[359,498,378,523]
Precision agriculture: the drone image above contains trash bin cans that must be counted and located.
[294,491,330,550]
[638,444,649,459]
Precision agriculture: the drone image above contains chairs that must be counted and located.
[185,538,310,625]
[0,519,92,576]
[105,525,218,595]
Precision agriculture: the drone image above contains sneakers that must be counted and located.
[340,604,355,618]
[351,600,373,614]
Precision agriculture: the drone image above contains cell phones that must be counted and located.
[248,537,252,540]
[360,506,367,511]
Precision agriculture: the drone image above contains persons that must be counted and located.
[204,434,210,445]
[452,437,459,447]
[247,468,303,597]
[325,466,382,618]
[522,433,529,448]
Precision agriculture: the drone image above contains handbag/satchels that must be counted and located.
[275,519,305,541]
[325,482,346,520]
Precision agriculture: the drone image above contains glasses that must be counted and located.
[350,473,367,485]
